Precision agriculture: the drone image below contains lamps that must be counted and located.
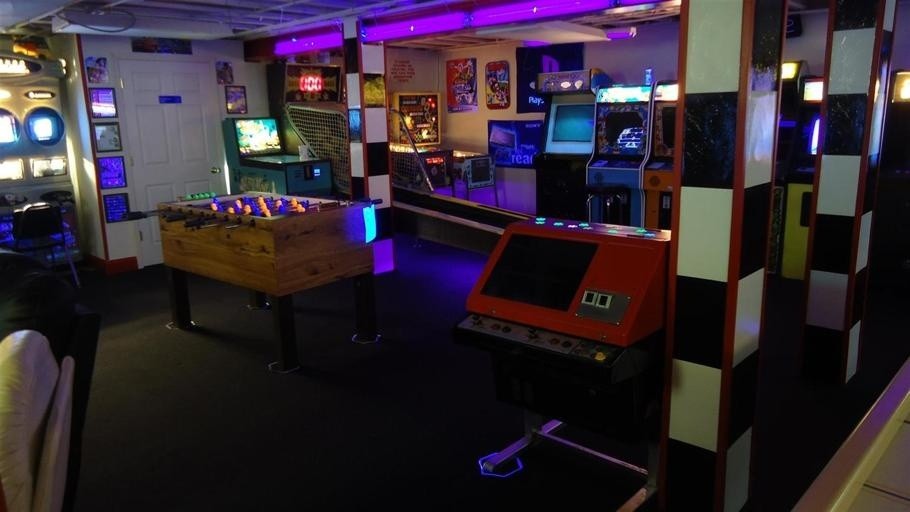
[781,58,811,119]
[888,69,910,104]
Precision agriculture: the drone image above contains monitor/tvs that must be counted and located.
[481,233,603,314]
[549,86,820,174]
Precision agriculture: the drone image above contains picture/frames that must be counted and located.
[97,155,127,189]
[88,87,118,118]
[93,122,124,152]
[103,193,129,223]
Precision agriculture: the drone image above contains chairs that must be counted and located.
[13,202,82,288]
[0,329,76,512]
[0,270,101,512]
